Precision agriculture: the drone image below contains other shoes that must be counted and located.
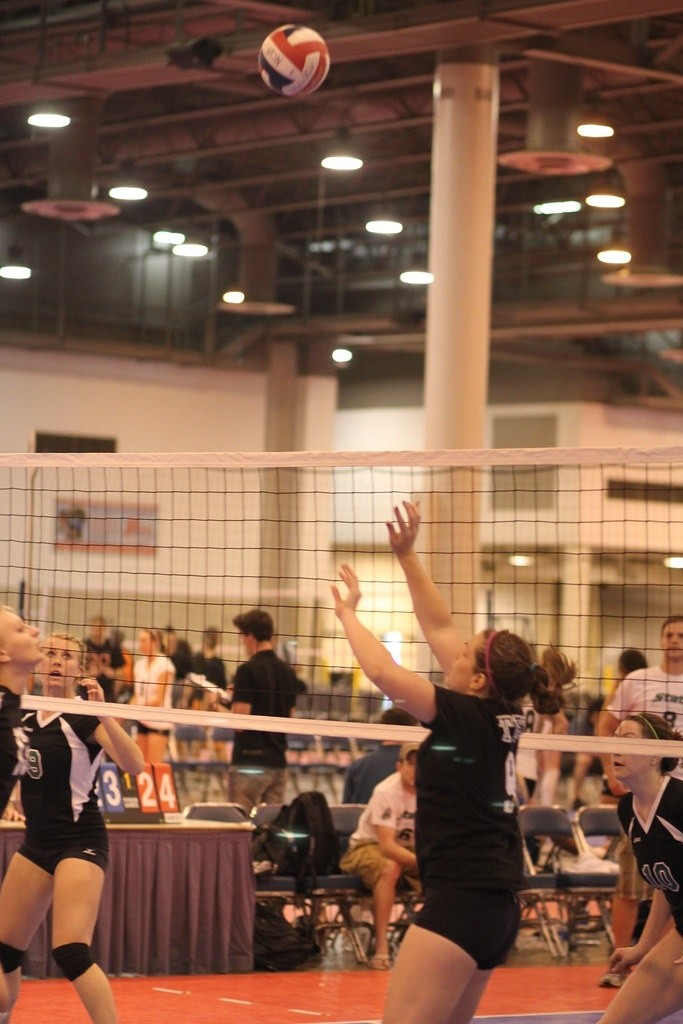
[368,945,390,970]
[598,969,633,988]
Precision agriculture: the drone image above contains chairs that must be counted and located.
[165,725,620,966]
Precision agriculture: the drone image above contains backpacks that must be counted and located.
[262,790,339,896]
[255,898,321,971]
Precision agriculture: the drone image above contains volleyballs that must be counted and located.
[255,23,332,97]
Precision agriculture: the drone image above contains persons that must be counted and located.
[0,604,46,818]
[332,501,566,1023]
[0,630,146,1024]
[77,610,296,818]
[340,613,683,1024]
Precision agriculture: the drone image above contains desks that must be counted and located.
[0,821,257,975]
